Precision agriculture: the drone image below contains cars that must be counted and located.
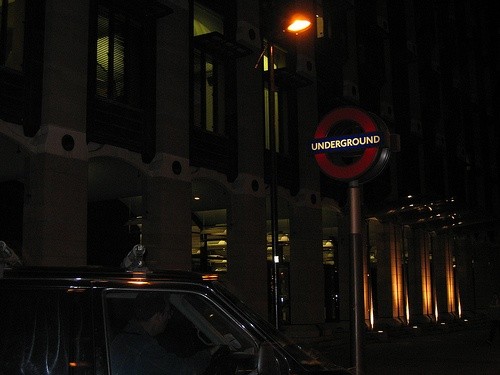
[1,265,351,374]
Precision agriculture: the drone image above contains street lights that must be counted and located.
[261,11,314,332]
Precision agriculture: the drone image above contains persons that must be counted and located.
[111,291,234,375]
